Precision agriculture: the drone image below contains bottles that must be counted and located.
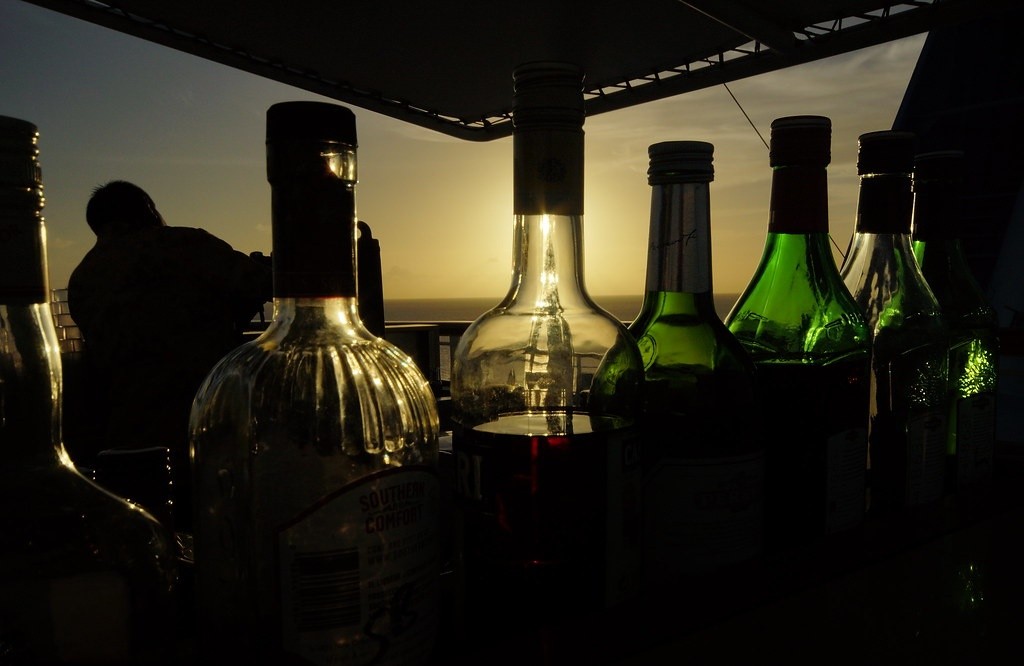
[937,239,999,507]
[452,61,644,613]
[727,116,872,546]
[588,141,762,601]
[185,101,440,666]
[838,131,947,537]
[0,116,183,666]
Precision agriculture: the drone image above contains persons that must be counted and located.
[69,181,272,446]
